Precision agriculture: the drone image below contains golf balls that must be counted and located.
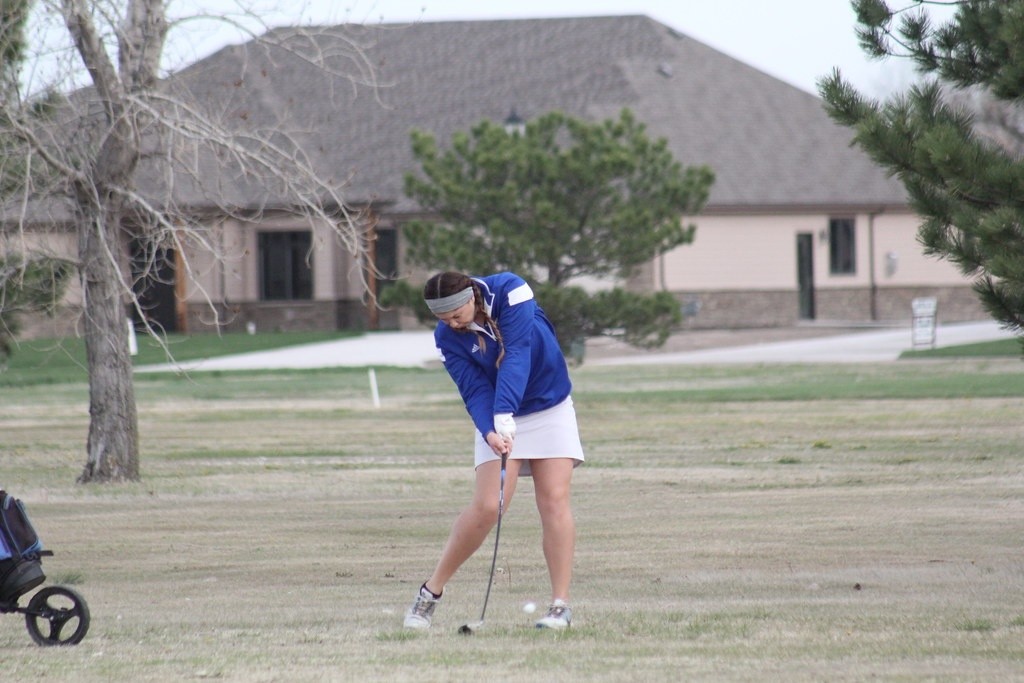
[523,602,536,614]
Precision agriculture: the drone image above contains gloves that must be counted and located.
[494,412,517,442]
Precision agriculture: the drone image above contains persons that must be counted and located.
[404,272,585,631]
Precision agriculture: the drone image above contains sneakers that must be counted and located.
[403,580,443,629]
[536,598,574,630]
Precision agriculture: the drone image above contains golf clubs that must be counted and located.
[457,452,507,634]
